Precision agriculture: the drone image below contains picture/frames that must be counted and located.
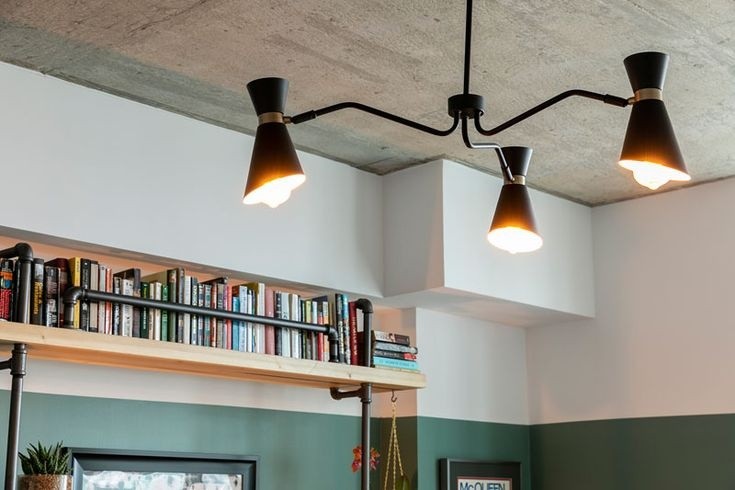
[60,447,260,490]
[441,458,524,490]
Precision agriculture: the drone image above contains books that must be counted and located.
[0,252,20,323]
[72,257,359,365]
[370,330,419,370]
[31,255,70,328]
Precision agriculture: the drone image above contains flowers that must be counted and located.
[352,445,381,472]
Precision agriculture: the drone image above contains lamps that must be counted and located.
[244,0,691,253]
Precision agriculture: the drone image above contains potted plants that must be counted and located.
[17,440,74,490]
